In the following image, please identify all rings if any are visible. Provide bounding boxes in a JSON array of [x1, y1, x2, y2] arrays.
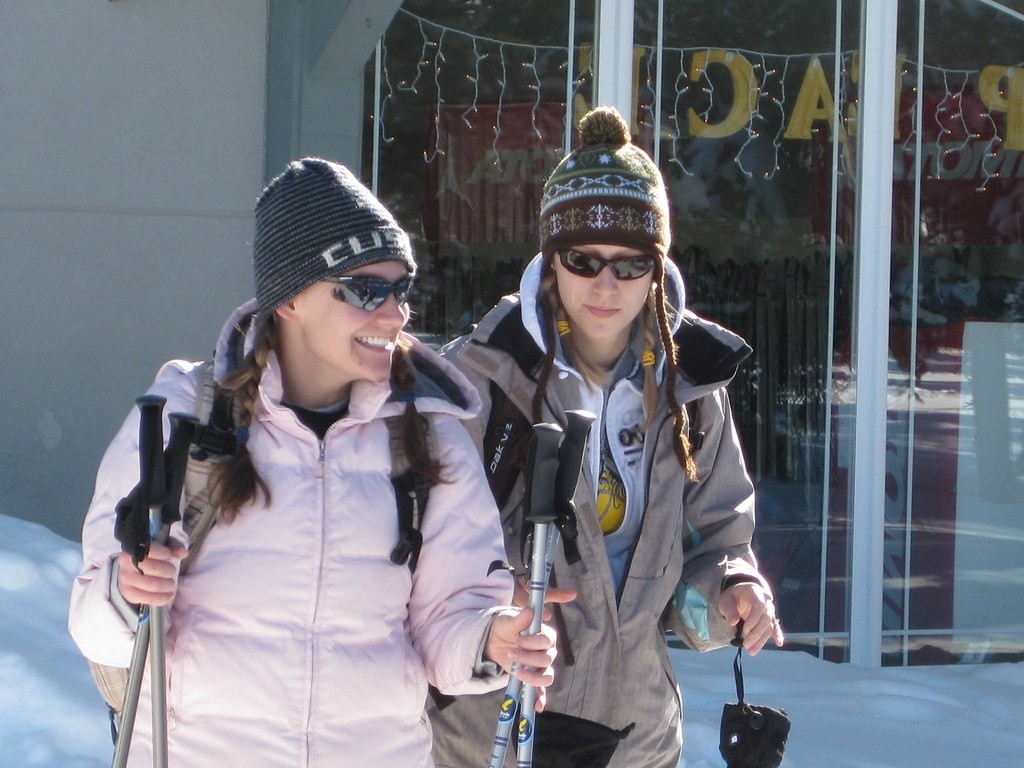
[[771, 616, 780, 630]]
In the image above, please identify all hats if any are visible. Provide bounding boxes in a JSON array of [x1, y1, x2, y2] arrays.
[[505, 106, 698, 482], [253, 156, 417, 317]]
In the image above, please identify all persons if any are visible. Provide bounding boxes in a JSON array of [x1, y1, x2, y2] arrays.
[[69, 157, 558, 768], [428, 106, 785, 768]]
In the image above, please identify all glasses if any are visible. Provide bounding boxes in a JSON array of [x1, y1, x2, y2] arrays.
[[320, 274, 412, 311], [558, 248, 655, 281]]
